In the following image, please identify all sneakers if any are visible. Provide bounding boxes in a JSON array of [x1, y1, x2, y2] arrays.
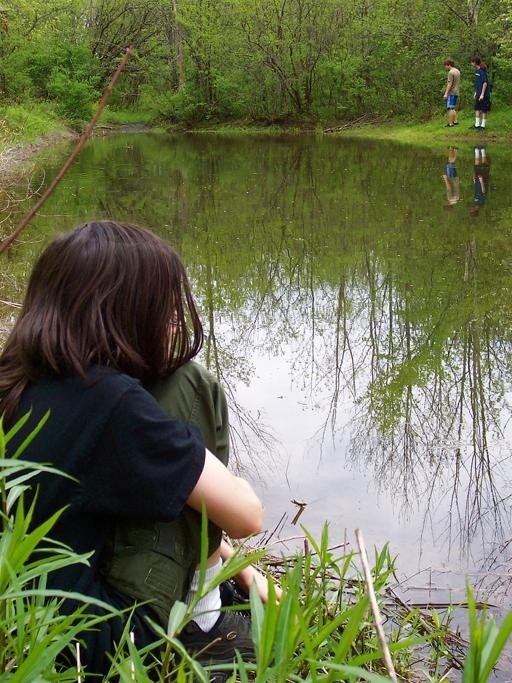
[[468, 125, 486, 131], [444, 121, 459, 128], [179, 579, 300, 677]]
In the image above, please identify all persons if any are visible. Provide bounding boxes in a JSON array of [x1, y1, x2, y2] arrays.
[[470, 145, 491, 217], [469, 55, 490, 130], [444, 60, 461, 128], [442, 144, 461, 211], [1, 219, 289, 683]]
